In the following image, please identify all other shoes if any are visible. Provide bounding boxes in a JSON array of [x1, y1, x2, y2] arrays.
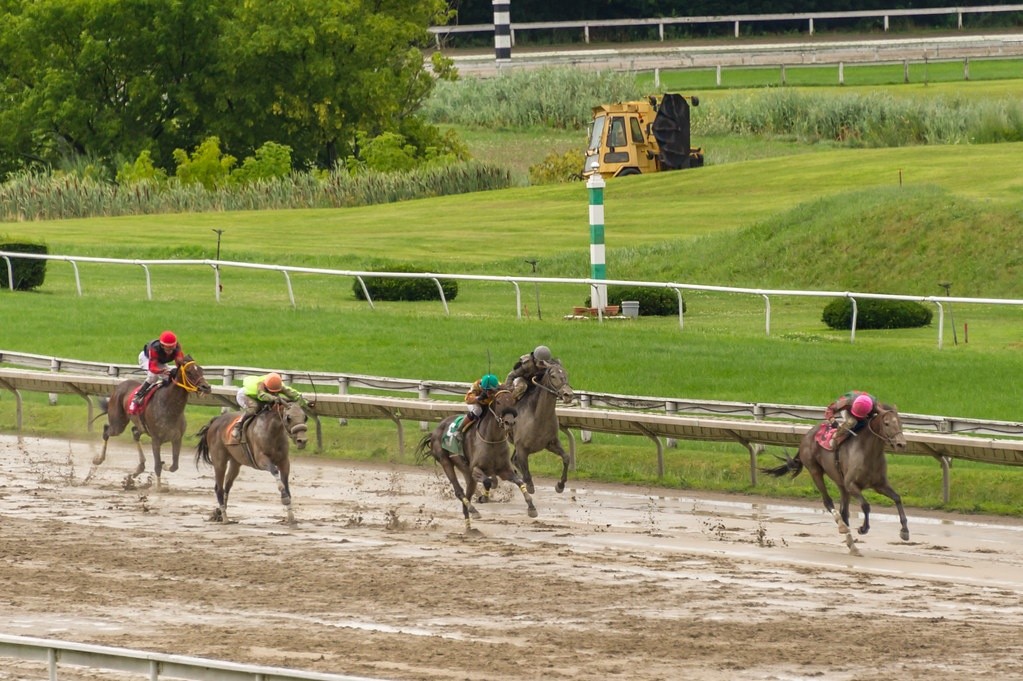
[[453, 431, 464, 442], [829, 438, 837, 451], [134, 394, 140, 404], [232, 428, 241, 440]]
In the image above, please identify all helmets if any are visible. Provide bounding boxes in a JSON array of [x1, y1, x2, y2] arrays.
[[852, 395, 872, 417], [481, 374, 498, 389], [160, 331, 177, 347], [264, 373, 283, 393]]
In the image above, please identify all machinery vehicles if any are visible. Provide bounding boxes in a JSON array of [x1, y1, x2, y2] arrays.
[[576, 93, 705, 182]]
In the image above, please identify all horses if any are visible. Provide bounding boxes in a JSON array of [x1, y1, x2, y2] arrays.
[[90, 354, 211, 492], [414, 384, 539, 520], [192, 396, 309, 524], [759, 402, 910, 540], [479, 357, 574, 504]]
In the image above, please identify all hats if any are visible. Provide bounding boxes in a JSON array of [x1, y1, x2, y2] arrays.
[[533, 346, 552, 363]]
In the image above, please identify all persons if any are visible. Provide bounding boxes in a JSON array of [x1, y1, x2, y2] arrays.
[[506, 345, 551, 402], [452, 374, 510, 440], [233, 372, 311, 439], [131, 330, 185, 405], [824, 389, 879, 450]]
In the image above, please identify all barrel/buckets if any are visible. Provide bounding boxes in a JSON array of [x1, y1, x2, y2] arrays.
[[622, 300, 640, 318]]
